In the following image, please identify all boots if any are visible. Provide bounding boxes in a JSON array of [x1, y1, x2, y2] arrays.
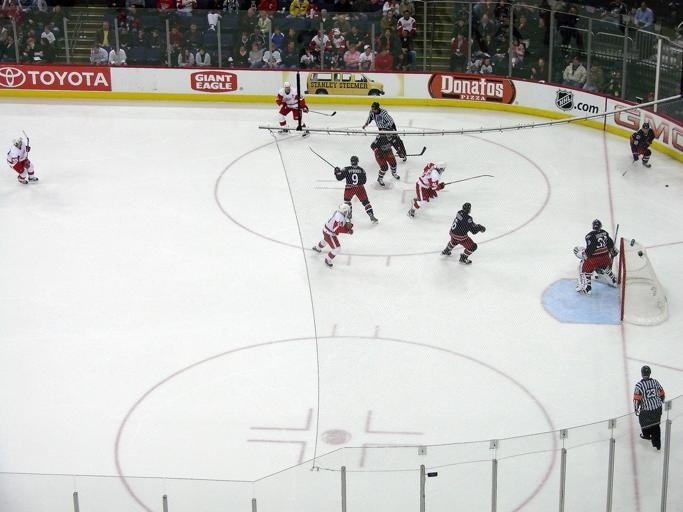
[[576, 283, 591, 294], [441, 247, 451, 255], [407, 208, 414, 217], [458, 253, 472, 263]]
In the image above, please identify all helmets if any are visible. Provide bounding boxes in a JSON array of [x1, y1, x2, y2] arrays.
[[642, 122, 649, 129], [641, 365, 651, 376], [338, 201, 351, 217], [283, 82, 290, 88], [434, 161, 447, 169], [349, 156, 358, 163], [462, 202, 471, 212], [10, 135, 23, 146], [592, 219, 601, 227]]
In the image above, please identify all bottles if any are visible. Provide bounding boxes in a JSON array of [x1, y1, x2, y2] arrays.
[[228, 56, 234, 68]]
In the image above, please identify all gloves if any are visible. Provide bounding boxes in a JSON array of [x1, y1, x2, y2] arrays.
[[633, 154, 638, 161], [302, 107, 309, 113], [573, 247, 588, 261], [279, 102, 286, 109], [25, 146, 30, 152], [438, 182, 444, 189], [334, 167, 341, 174]]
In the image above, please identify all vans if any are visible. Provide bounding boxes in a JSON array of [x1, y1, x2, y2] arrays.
[[305, 72, 385, 97]]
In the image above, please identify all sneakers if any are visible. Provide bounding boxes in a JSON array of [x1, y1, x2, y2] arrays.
[[27, 176, 37, 181], [312, 246, 321, 252], [369, 215, 377, 222], [377, 157, 406, 186], [642, 161, 651, 167], [278, 129, 288, 133], [302, 125, 308, 137], [17, 176, 27, 184], [324, 257, 333, 266], [640, 433, 650, 439]]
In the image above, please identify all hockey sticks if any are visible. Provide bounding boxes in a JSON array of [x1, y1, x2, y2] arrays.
[[591, 224, 619, 280], [22, 131, 29, 158], [444, 175, 493, 184], [622, 160, 636, 175], [394, 147, 426, 156], [308, 110, 336, 116], [306, 144, 335, 168]]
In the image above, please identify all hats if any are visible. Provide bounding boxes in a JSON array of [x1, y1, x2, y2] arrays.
[[371, 102, 379, 108]]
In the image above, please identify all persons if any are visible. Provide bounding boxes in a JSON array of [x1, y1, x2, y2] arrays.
[[405, 160, 445, 221], [574, 220, 617, 294], [628, 122, 655, 170], [632, 364, 665, 453], [5, 136, 38, 185], [440, 202, 486, 265], [87, 1, 418, 69], [368, 127, 400, 188], [275, 82, 310, 136], [0, 1, 72, 60], [332, 156, 378, 223], [361, 102, 407, 163], [447, 1, 681, 99], [311, 204, 350, 268]]
